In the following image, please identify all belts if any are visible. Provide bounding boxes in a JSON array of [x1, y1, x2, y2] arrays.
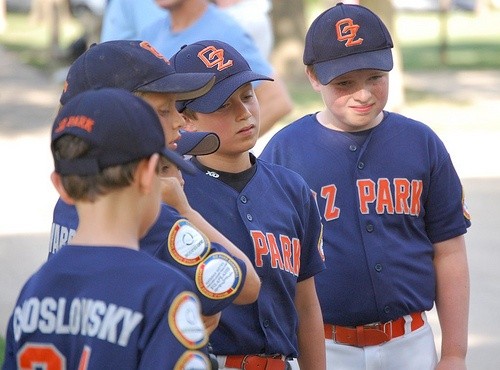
[[225, 354, 286, 370], [324, 310, 424, 346]]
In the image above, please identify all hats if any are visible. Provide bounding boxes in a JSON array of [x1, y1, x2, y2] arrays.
[[51, 85, 198, 177], [169, 39, 274, 113], [60, 40, 216, 105], [303, 2, 394, 86], [174, 127, 220, 156]]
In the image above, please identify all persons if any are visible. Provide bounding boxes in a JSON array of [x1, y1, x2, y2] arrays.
[[167, 38, 327, 370], [135, 0, 293, 139], [98, 0, 275, 61], [46, 39, 261, 317], [1, 87, 211, 370], [257, 2, 472, 370], [160, 152, 222, 336]]
